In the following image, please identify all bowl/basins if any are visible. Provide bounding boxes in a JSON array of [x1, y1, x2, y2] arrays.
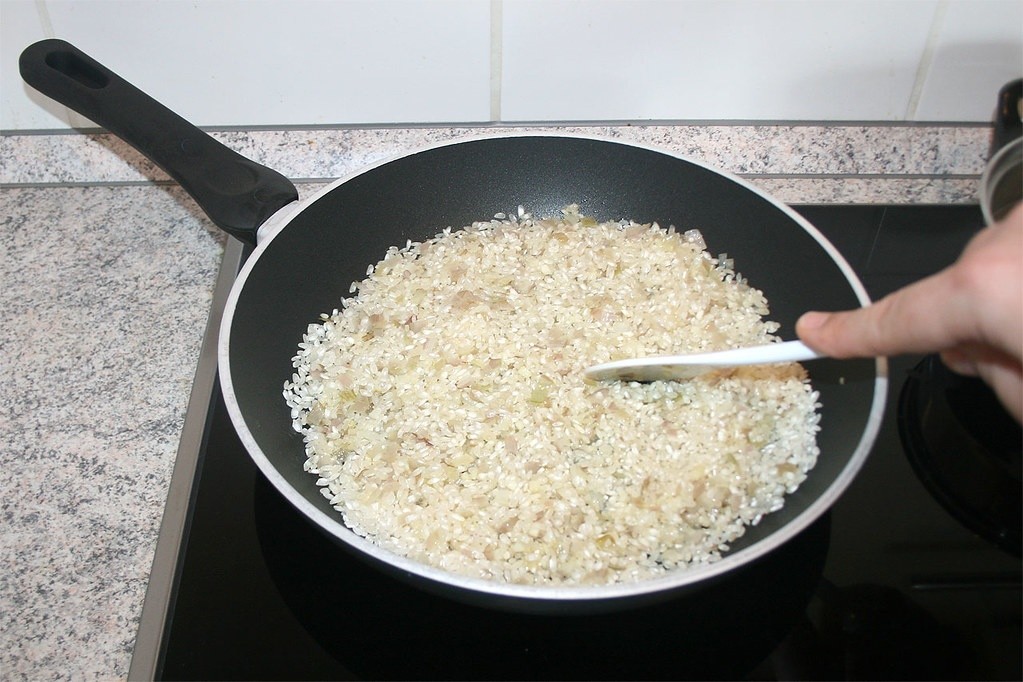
[[981, 136, 1023, 228]]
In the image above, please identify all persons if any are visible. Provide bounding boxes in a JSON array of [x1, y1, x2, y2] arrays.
[[795, 199, 1023, 424]]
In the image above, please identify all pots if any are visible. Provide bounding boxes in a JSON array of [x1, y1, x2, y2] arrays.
[[17, 37, 889, 619]]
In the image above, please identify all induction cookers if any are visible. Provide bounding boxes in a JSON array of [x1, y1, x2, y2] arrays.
[[126, 202, 1022, 682]]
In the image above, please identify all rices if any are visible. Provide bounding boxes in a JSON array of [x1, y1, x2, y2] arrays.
[[280, 197, 828, 586]]
